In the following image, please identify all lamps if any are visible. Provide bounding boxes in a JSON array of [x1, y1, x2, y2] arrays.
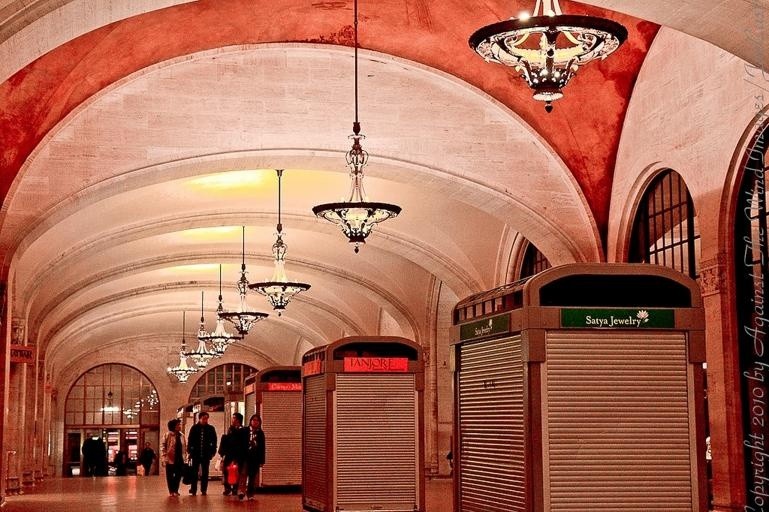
[[166, 166, 311, 383], [466, 1, 627, 115], [312, 2, 404, 254]]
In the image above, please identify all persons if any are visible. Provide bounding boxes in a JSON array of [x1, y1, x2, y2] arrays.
[[218, 411, 245, 496], [159, 419, 190, 497], [114, 445, 129, 476], [186, 411, 218, 496], [139, 442, 158, 476], [231, 414, 266, 501]]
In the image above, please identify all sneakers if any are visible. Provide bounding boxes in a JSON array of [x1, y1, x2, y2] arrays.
[[170, 489, 206, 496], [223, 490, 255, 500]]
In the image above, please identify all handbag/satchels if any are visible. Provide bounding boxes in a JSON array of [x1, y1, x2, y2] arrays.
[[227, 461, 239, 486], [183, 466, 191, 486], [180, 464, 188, 475], [218, 435, 225, 456]]
